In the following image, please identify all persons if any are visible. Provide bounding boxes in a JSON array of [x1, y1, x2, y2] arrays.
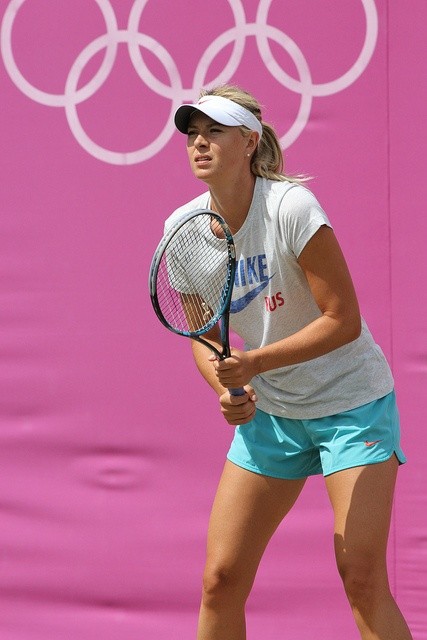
[[161, 80, 417, 639]]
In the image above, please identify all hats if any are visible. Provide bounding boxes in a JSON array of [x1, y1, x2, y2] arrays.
[[175, 95, 263, 143]]
